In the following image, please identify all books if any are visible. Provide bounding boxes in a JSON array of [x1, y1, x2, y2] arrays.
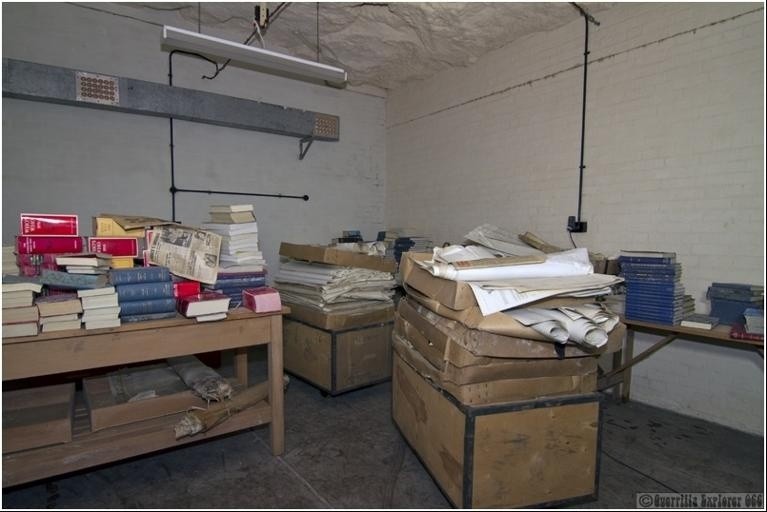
[[378, 230, 434, 272], [679, 313, 720, 331], [327, 230, 364, 247], [707, 281, 764, 336], [592, 248, 696, 326], [2, 203, 282, 338]]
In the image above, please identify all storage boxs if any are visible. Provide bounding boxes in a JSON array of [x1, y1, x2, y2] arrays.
[[283, 316, 394, 397], [389, 350, 604, 509]]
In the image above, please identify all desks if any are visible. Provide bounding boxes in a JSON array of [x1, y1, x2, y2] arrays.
[[598, 314, 764, 404], [2, 306, 292, 490]]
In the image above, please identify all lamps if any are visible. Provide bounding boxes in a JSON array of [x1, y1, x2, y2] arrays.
[[160, 24, 348, 85]]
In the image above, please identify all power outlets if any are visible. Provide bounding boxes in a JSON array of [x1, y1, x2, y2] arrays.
[[567, 222, 587, 232]]
